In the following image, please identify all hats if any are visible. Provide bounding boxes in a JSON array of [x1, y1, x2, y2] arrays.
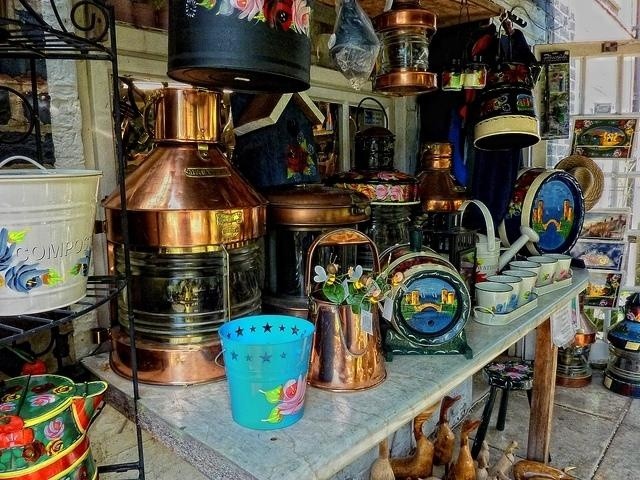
[[554, 155, 605, 213]]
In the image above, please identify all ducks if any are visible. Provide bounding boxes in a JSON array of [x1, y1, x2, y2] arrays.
[[370, 397, 483, 480]]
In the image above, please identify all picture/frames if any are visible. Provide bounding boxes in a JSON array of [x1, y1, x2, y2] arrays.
[[583, 273, 623, 310], [578, 206, 631, 241], [571, 238, 628, 273], [568, 115, 639, 157]]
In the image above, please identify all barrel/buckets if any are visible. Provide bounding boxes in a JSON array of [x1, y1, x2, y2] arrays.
[[0, 155, 103, 317], [373, 245, 473, 347], [215, 315, 316, 430], [102, 145, 269, 386]]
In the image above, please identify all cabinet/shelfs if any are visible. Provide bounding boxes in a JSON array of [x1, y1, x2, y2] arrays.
[[570, 130, 640, 342], [0, 0, 145, 480]]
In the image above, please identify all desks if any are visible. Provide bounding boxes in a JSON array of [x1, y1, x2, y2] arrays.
[[78, 268, 590, 480]]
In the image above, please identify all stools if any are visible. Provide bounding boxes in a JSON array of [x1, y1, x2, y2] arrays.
[[471, 359, 552, 463]]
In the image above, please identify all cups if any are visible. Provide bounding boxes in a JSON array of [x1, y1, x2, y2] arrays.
[[474, 253, 573, 314]]
